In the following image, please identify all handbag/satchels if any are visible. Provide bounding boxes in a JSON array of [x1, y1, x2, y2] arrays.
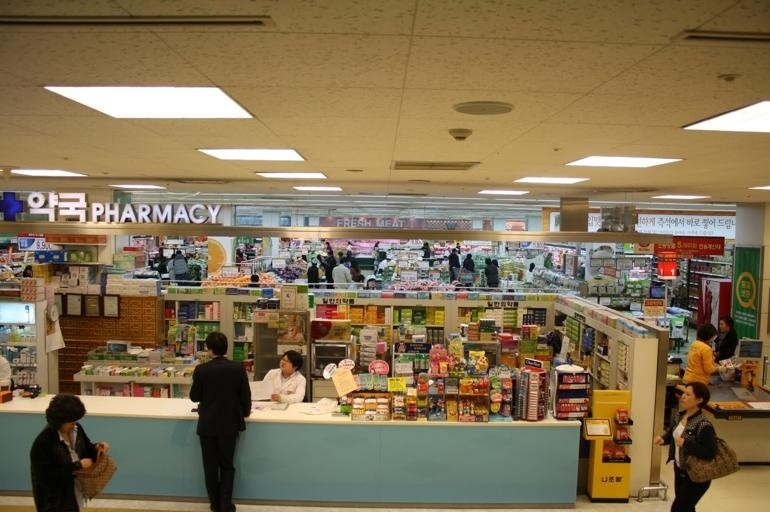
[[73, 448, 117, 500], [686, 419, 740, 483]]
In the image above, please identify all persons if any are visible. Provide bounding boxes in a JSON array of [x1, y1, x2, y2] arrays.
[[0, 357, 12, 393], [525, 263, 544, 285], [301, 240, 500, 291], [714, 316, 738, 365], [248, 350, 308, 404], [237, 242, 260, 287], [544, 252, 554, 271], [29, 393, 110, 512], [654, 381, 717, 512], [149, 242, 199, 286], [190, 331, 252, 512], [680, 322, 729, 389]]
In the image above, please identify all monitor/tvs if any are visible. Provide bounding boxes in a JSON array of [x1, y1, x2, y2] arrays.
[[735, 337, 763, 362], [650, 286, 665, 299]]
